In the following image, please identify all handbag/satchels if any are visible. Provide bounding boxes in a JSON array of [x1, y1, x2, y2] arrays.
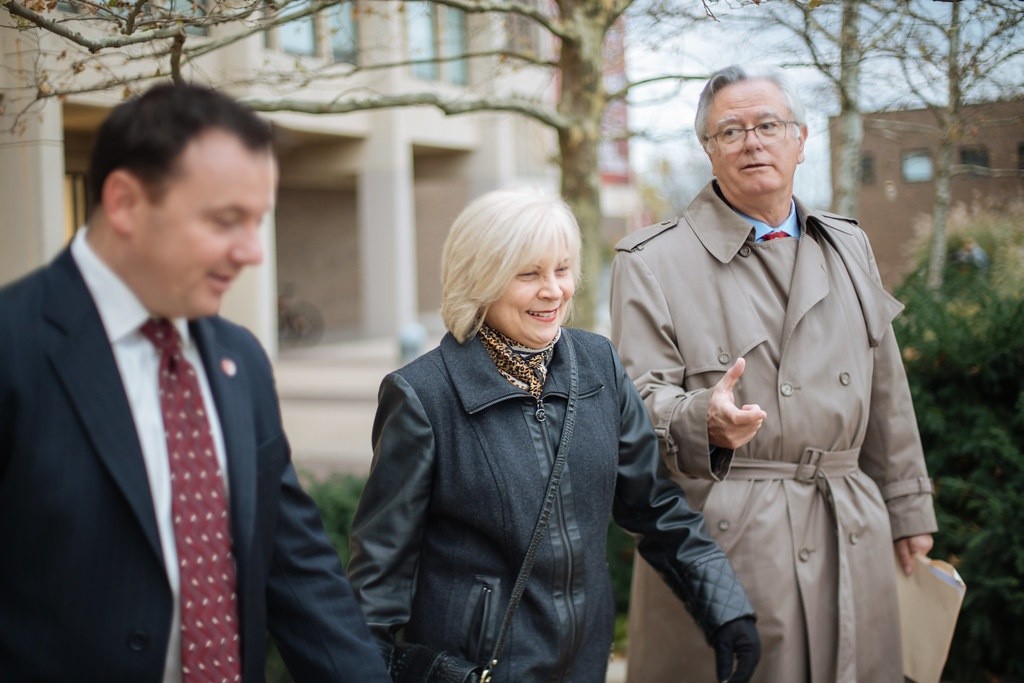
[[386, 641, 490, 683]]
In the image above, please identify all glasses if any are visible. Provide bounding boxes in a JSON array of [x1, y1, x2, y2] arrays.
[[707, 119, 800, 154]]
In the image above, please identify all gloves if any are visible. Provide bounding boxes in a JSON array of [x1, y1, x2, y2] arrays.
[[713, 612, 762, 682]]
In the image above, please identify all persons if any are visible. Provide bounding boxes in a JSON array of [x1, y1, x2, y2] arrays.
[[347, 184, 763, 682], [612, 67, 937, 683], [2, 80, 392, 683]]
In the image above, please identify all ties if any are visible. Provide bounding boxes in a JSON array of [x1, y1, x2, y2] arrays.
[[142, 318, 243, 682]]
